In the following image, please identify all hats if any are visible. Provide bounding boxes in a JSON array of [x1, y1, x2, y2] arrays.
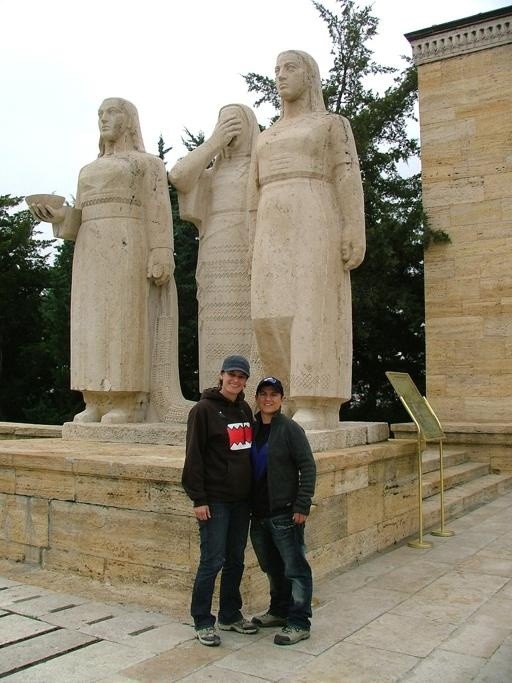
[[256, 377, 283, 395], [221, 355, 250, 377]]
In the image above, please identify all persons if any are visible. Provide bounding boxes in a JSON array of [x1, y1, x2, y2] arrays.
[[180, 354, 259, 646], [250, 375, 317, 645], [168, 103, 264, 393], [23, 96, 178, 424], [246, 49, 367, 428]]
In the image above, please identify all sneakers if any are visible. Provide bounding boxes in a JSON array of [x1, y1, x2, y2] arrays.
[[274, 624, 310, 645], [195, 626, 220, 646], [217, 618, 259, 634], [251, 613, 289, 627]]
